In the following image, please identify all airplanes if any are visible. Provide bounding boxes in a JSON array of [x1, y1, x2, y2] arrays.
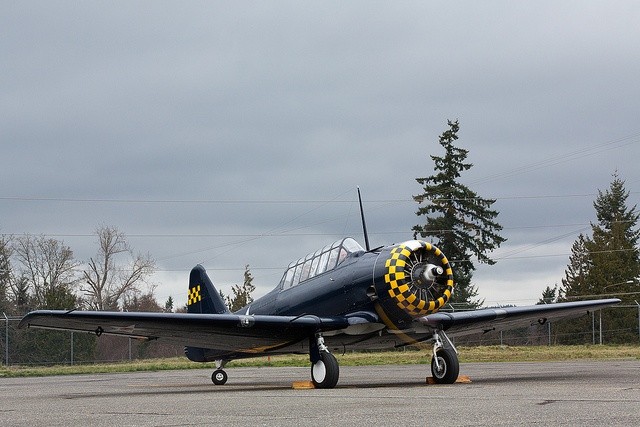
[[19, 188, 621, 388]]
